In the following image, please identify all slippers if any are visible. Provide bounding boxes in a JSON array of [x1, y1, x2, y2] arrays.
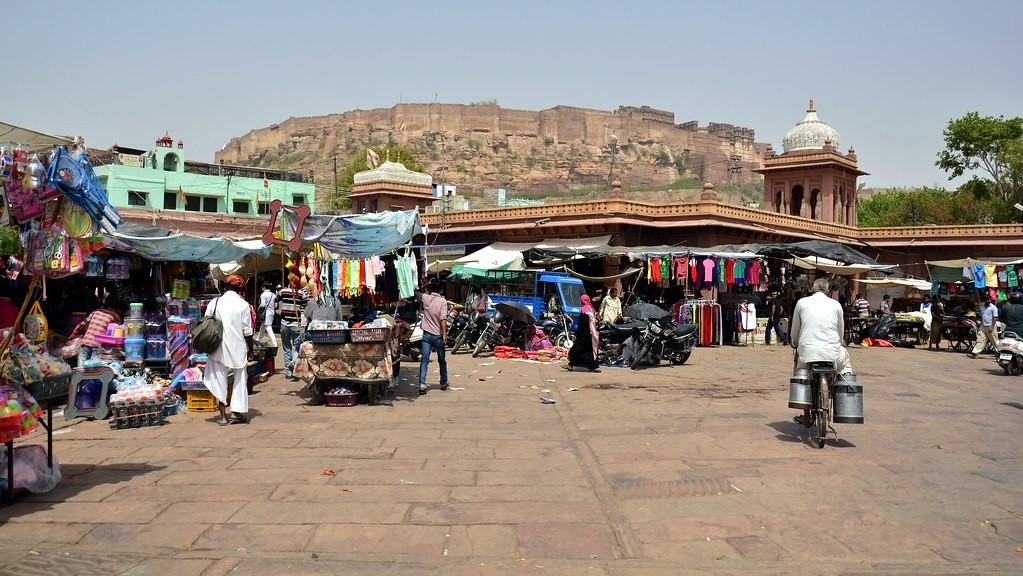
[[228, 415, 247, 421], [216, 418, 228, 426], [794, 415, 811, 429]]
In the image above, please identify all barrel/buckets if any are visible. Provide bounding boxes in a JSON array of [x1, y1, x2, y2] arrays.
[[130, 303, 143, 319], [788, 367, 813, 410], [832, 372, 864, 424]]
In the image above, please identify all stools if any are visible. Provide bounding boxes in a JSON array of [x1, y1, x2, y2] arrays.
[[108, 399, 163, 429]]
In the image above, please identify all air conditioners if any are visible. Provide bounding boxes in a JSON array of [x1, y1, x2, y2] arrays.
[[426, 204, 439, 213]]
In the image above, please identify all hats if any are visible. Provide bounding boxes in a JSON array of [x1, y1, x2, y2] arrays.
[[224, 274, 245, 290]]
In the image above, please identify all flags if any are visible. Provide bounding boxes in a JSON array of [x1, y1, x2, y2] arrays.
[[366, 148, 380, 170], [396, 117, 406, 136]]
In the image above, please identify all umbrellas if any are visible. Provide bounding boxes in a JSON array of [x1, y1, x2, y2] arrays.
[[495, 300, 537, 336], [623, 303, 671, 320]]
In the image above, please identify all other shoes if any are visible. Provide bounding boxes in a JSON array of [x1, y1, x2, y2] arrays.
[[561, 365, 573, 371], [966, 353, 977, 359], [783, 338, 789, 346], [288, 362, 300, 381], [286, 375, 293, 379], [589, 369, 602, 373], [441, 380, 450, 390], [419, 386, 431, 395], [760, 342, 772, 346]]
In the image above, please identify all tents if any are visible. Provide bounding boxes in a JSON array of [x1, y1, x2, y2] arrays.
[[429, 234, 1023, 315]]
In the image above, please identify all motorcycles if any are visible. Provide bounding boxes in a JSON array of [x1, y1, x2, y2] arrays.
[[631, 315, 699, 370], [402, 302, 576, 357]]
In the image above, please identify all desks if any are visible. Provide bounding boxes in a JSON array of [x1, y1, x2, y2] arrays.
[[5, 391, 68, 505], [121, 359, 172, 379]]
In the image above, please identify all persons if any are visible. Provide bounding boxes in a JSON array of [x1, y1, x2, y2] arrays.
[[201, 274, 253, 426], [348, 286, 377, 328], [66, 280, 128, 368], [940, 296, 971, 318], [275, 284, 281, 295], [852, 292, 871, 329], [763, 292, 790, 346], [0, 284, 20, 335], [560, 294, 601, 373], [620, 327, 652, 368], [919, 294, 947, 351], [468, 286, 480, 309], [880, 295, 891, 318], [966, 294, 1023, 359], [256, 282, 278, 346], [548, 296, 560, 315], [598, 288, 623, 325], [413, 281, 450, 395], [790, 277, 852, 428], [527, 323, 553, 351], [275, 282, 343, 380]]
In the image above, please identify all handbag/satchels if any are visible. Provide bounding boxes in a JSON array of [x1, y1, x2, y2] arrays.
[[24, 301, 48, 342], [191, 315, 223, 355], [256, 306, 266, 322], [26, 219, 84, 279]]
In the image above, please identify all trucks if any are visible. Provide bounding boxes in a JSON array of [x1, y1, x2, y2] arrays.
[[484, 268, 586, 333]]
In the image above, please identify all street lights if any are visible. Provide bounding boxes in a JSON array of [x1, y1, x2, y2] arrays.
[[855, 181, 867, 228], [609, 135, 618, 191]]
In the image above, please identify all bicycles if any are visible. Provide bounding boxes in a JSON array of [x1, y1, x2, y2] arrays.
[[791, 343, 848, 448]]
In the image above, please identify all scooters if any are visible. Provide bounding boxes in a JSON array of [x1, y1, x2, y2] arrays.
[[994, 330, 1023, 376]]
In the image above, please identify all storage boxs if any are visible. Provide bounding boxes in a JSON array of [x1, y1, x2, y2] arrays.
[[324, 385, 367, 406], [94, 334, 125, 345], [347, 327, 390, 342], [164, 345, 278, 414], [307, 328, 349, 344], [20, 372, 76, 401]]
